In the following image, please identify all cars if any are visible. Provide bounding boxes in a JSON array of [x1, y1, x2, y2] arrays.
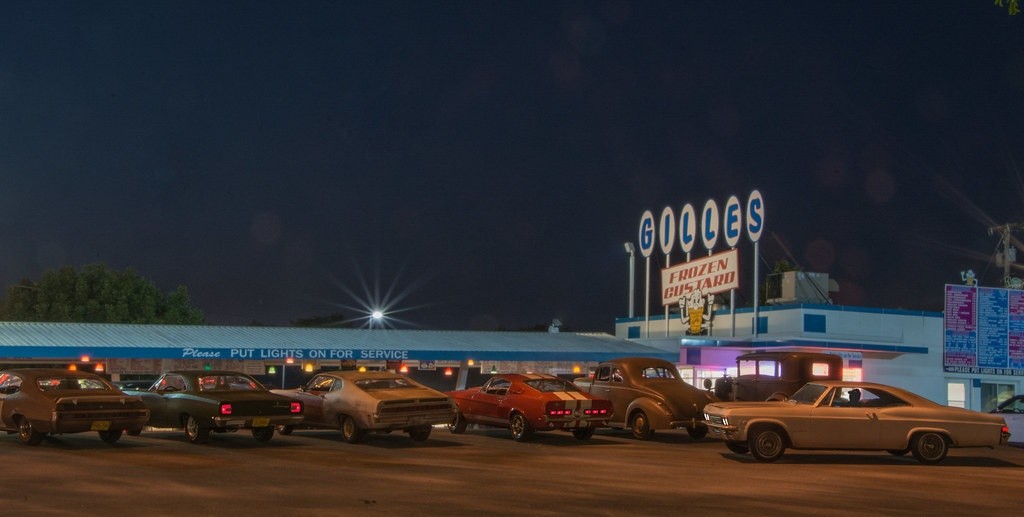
[[703, 380, 1013, 465], [0, 366, 151, 445], [443, 373, 615, 443], [271, 371, 460, 443], [120, 370, 305, 444], [703, 351, 844, 401], [985, 396, 1024, 448], [573, 357, 720, 441]]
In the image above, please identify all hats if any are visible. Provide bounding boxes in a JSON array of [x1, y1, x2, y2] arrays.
[[848, 389, 861, 397]]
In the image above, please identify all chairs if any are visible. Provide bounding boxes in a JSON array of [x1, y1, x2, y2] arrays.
[[216, 383, 231, 391], [357, 381, 390, 389], [58, 379, 80, 389]]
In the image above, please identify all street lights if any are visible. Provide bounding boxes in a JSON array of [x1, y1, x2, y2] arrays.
[[369, 310, 383, 330]]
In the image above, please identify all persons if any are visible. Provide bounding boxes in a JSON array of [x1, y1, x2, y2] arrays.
[[842, 389, 861, 406]]
